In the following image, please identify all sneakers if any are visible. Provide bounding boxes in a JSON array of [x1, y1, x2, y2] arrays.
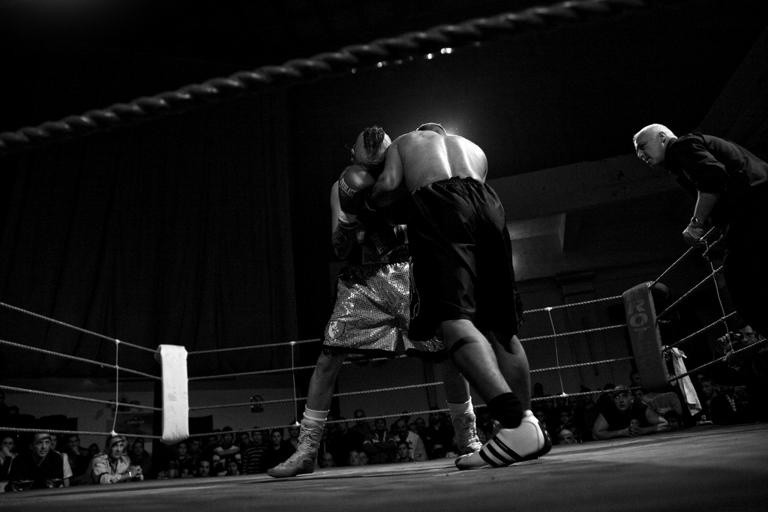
[[453, 408, 553, 470]]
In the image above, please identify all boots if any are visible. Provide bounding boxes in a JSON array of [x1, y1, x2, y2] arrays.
[[450, 409, 484, 456], [266, 420, 325, 478]]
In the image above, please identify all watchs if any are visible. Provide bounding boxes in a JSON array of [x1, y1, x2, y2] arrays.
[[690, 217, 707, 230]]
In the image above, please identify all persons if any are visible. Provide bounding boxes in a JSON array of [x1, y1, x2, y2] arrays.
[[0, 365, 767, 490], [267, 130, 483, 476], [633, 125, 768, 340], [374, 122, 553, 466]]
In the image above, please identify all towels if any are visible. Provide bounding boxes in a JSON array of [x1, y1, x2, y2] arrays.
[[670, 346, 703, 416]]
